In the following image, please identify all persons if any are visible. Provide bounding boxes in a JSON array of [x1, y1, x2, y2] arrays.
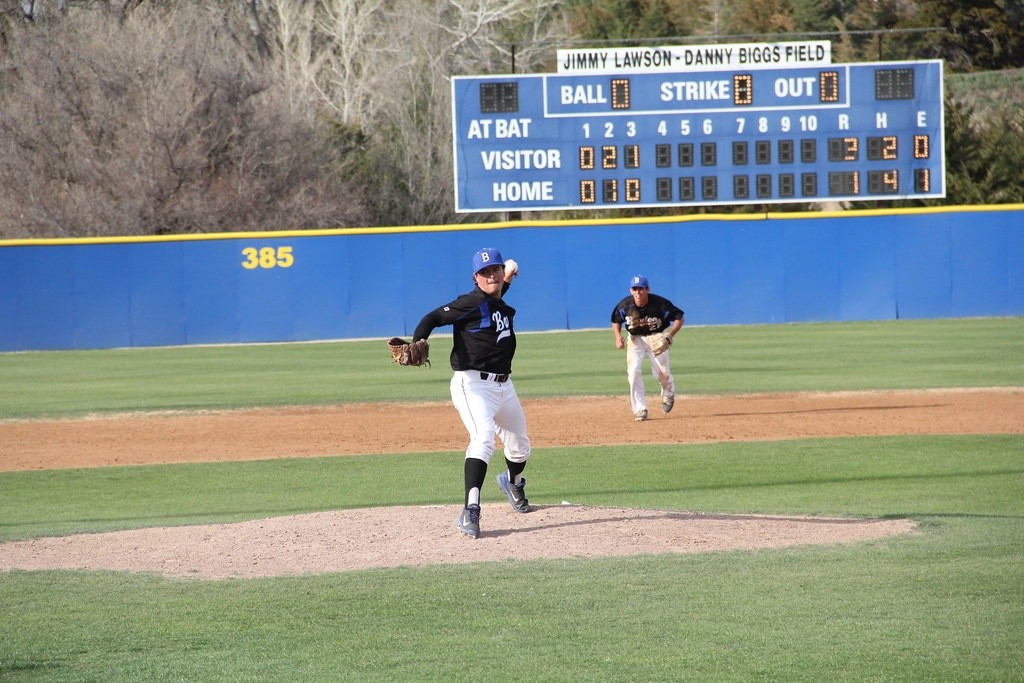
[[611, 273, 684, 422], [387, 248, 531, 539]]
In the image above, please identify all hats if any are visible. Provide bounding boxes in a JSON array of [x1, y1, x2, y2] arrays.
[[630, 274, 650, 288], [472, 247, 506, 275]]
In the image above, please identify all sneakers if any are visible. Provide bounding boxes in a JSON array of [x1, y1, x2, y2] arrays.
[[634, 409, 648, 421], [662, 395, 674, 412], [497, 469, 533, 514], [457, 502, 482, 539]]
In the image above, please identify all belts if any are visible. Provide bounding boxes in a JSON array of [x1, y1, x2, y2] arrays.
[[480, 372, 509, 382]]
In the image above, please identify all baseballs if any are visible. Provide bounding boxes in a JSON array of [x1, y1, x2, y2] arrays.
[[504, 260, 518, 274]]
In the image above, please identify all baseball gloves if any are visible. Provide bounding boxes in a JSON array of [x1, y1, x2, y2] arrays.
[[386, 337, 432, 371], [647, 332, 673, 357]]
